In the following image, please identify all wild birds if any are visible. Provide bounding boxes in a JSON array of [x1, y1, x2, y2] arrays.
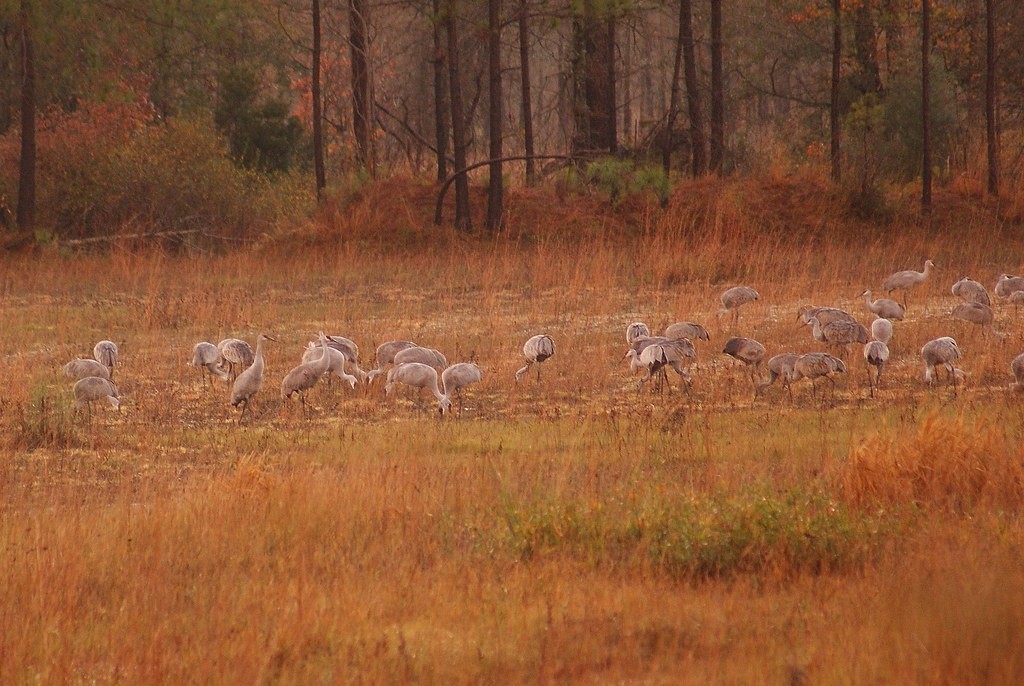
[[58, 340, 123, 428], [617, 321, 710, 405], [280, 329, 483, 423], [185, 333, 280, 425], [514, 333, 558, 386], [719, 259, 1024, 405]]
